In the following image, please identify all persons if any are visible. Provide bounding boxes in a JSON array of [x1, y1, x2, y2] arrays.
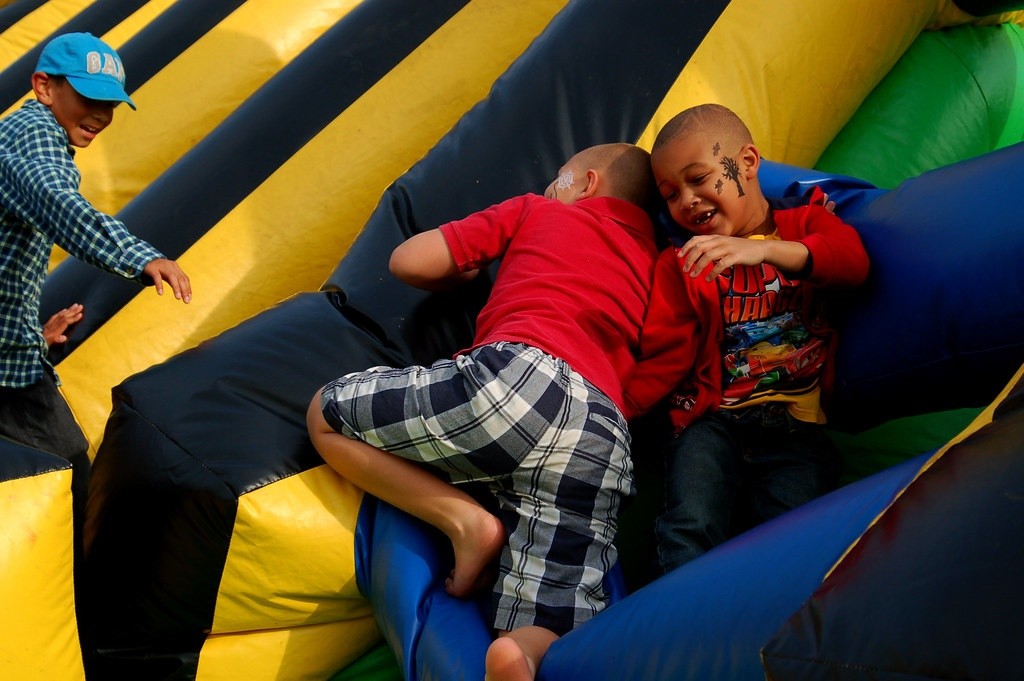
[[0, 32, 191, 550], [627, 103, 870, 569], [307, 143, 834, 681]]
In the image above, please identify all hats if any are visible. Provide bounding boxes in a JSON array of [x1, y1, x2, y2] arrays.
[[36, 32, 137, 111]]
